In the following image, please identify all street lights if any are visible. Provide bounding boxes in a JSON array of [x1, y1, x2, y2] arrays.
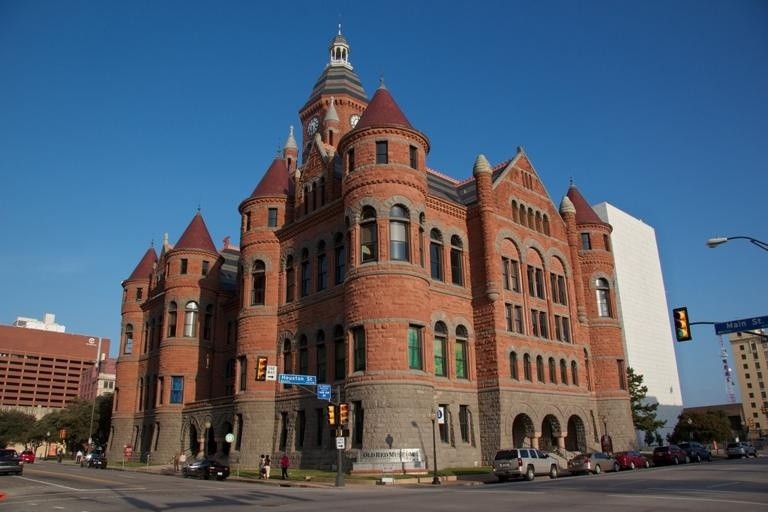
[[430, 409, 441, 485], [601, 415, 608, 456], [707, 236, 768, 251], [688, 418, 693, 439]]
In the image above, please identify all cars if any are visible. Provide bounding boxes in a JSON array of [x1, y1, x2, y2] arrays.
[[618, 449, 653, 469], [184, 461, 229, 480], [20, 451, 35, 464], [0, 449, 23, 476], [80, 454, 106, 470], [567, 452, 620, 474]]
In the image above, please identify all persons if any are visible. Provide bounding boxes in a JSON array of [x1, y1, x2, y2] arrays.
[[175, 451, 180, 471], [280, 453, 289, 479], [180, 452, 186, 468], [259, 454, 272, 480], [199, 455, 208, 479], [56, 445, 82, 463]]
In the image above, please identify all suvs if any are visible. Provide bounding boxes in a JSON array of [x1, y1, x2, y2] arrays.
[[492, 448, 559, 481]]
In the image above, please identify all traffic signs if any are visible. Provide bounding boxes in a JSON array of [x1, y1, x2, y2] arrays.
[[278, 374, 317, 386], [715, 316, 768, 335]]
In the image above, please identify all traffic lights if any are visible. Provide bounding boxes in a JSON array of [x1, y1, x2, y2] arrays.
[[256, 358, 267, 380], [670, 308, 690, 342], [341, 403, 349, 425], [326, 406, 335, 425]]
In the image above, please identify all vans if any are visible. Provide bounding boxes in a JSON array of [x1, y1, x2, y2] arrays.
[[680, 441, 714, 462], [654, 445, 690, 465], [726, 441, 758, 459]]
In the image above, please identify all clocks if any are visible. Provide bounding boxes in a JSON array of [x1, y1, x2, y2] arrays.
[[348, 113, 361, 129], [306, 115, 320, 137]]
